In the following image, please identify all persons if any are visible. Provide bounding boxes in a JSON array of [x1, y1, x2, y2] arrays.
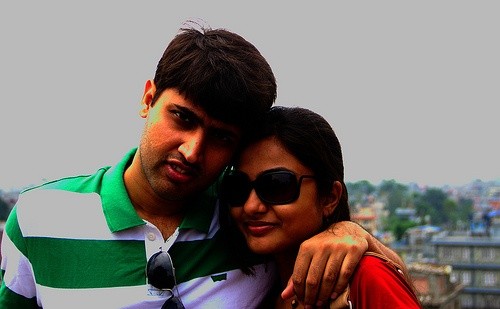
[[0, 16, 410, 309], [216, 106, 421, 309]]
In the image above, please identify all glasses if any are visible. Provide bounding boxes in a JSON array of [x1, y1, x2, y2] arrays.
[[147, 247, 185, 309], [220, 170, 317, 207]]
